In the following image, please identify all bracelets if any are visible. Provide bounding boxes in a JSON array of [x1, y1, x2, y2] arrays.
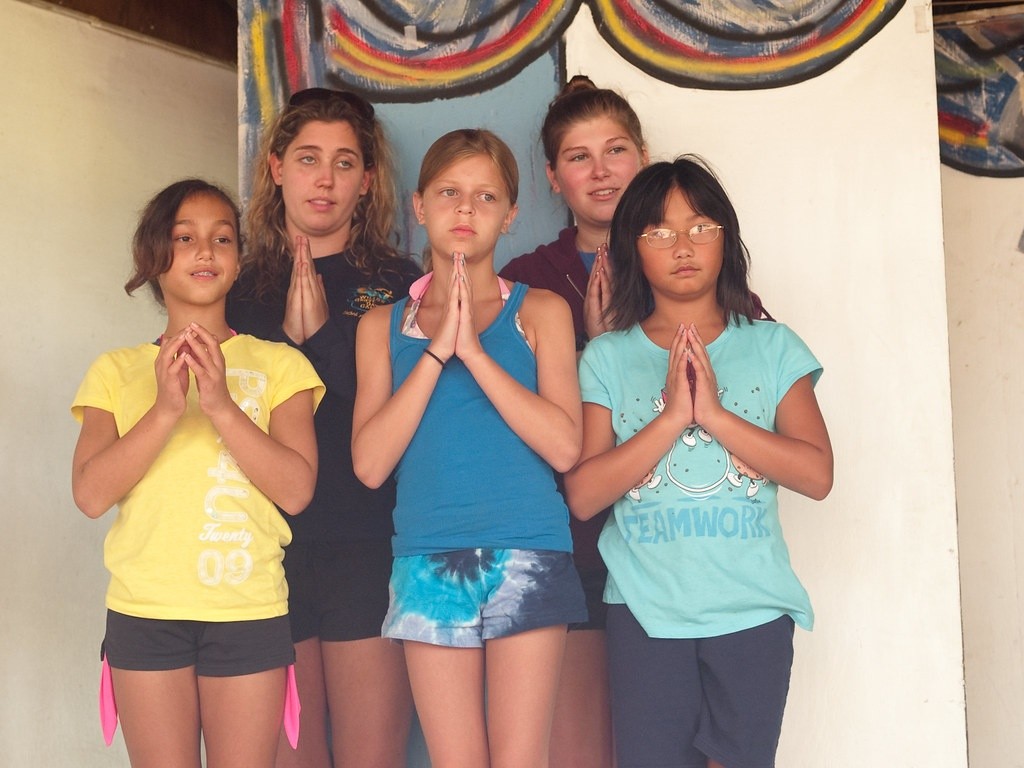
[[423, 349, 445, 369]]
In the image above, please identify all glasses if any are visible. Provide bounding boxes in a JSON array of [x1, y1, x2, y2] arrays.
[[636, 223, 724, 249]]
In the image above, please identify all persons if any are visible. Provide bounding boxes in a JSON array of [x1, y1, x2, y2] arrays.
[[351, 129, 589, 768], [495, 75, 762, 768], [69, 179, 327, 768], [562, 159, 834, 768], [225, 86, 425, 768]]
